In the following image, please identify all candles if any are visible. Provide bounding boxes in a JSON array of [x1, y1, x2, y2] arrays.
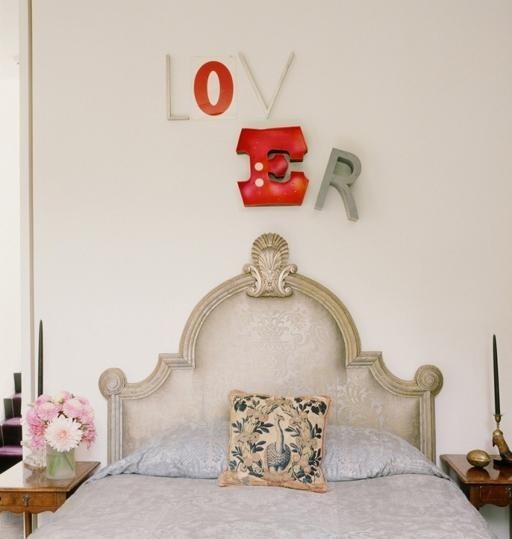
[[493, 334, 501, 416]]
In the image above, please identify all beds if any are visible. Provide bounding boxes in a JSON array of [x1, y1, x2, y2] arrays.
[[20, 232, 499, 539]]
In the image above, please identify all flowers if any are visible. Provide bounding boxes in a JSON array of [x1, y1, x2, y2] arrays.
[[26, 392, 97, 471]]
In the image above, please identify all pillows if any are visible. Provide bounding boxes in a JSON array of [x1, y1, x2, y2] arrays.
[[219, 388, 332, 495], [85, 420, 230, 481], [324, 424, 448, 480]]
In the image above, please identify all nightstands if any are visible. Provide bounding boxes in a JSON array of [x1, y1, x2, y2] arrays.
[[437, 454, 511, 539], [0, 456, 99, 539]]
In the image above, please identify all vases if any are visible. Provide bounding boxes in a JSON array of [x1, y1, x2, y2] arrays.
[[46, 445, 75, 480]]
